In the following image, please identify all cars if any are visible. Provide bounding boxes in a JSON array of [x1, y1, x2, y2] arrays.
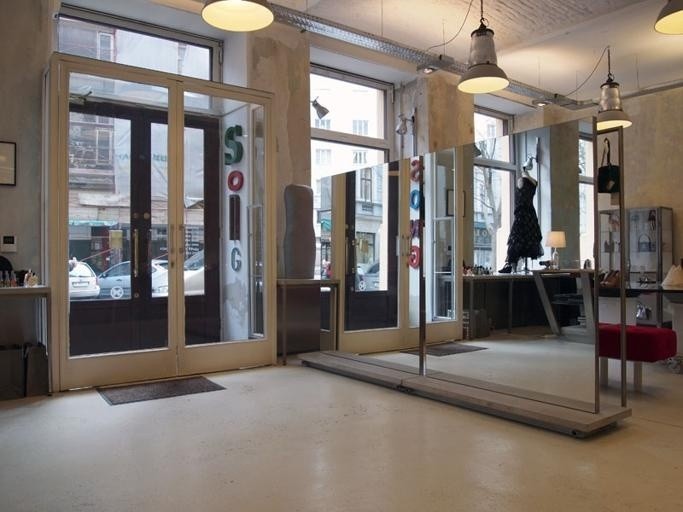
[[357, 261, 380, 291], [69, 249, 204, 297]]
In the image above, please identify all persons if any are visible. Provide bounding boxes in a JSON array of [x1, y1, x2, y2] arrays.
[[505, 169, 545, 264]]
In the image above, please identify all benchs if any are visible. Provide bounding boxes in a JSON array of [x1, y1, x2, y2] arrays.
[[599, 322, 677, 392]]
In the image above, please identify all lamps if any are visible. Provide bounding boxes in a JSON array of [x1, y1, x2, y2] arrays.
[[654, 0, 683, 36], [200, 0, 274, 33], [457, 0, 510, 95], [395, 113, 416, 136], [310, 99, 329, 119], [595, 45, 632, 132], [545, 231, 567, 270]]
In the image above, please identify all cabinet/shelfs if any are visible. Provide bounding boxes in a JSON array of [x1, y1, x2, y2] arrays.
[[599, 206, 675, 328]]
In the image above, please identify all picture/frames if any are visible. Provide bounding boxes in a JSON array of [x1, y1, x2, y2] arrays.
[[0, 140, 17, 186], [446, 190, 465, 218]]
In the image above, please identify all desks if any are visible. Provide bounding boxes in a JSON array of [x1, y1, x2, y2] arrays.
[[438, 272, 570, 341], [0, 283, 53, 397]]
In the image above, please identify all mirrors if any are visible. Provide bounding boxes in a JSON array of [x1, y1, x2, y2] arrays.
[[320, 116, 600, 413]]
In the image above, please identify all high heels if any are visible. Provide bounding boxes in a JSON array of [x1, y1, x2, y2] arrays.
[[599, 269, 620, 289]]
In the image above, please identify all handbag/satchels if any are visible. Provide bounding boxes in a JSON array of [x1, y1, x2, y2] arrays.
[[635, 301, 653, 321], [596, 160, 623, 194], [636, 233, 655, 252]]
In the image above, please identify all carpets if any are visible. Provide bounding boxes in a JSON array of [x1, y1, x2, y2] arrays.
[[400, 342, 488, 357], [95, 375, 227, 406]]
